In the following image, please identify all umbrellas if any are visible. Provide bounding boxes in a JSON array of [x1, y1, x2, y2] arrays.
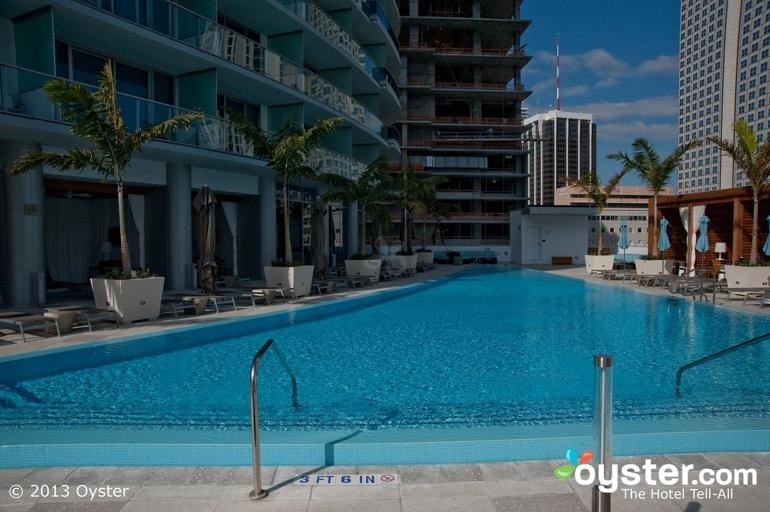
[[656, 216, 672, 274], [192, 184, 218, 297], [615, 222, 631, 273], [696, 215, 710, 267], [762, 212, 770, 256]]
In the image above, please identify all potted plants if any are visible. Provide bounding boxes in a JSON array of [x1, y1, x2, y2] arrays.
[[8, 58, 204, 326], [223, 102, 344, 300], [557, 116, 769, 296], [319, 146, 448, 285]]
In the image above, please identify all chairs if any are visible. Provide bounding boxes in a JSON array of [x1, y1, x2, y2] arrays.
[[722, 288, 770, 307], [312, 278, 348, 296], [1, 278, 298, 341]]
